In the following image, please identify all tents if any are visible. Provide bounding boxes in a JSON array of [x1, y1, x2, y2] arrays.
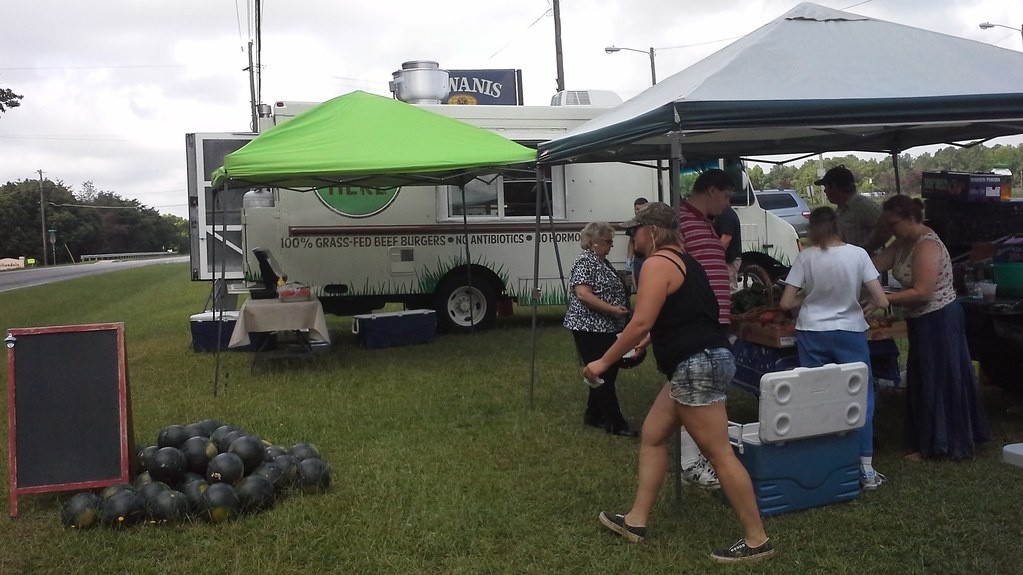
[[210, 90, 583, 399], [536, 1, 1022, 501]]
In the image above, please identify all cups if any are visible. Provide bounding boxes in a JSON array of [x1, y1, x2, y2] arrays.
[[982, 284, 997, 303]]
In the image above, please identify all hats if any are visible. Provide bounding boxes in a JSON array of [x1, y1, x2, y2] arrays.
[[814, 164, 854, 188], [619, 202, 677, 229]]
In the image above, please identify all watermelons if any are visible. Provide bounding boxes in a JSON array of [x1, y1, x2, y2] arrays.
[[60, 419, 328, 530]]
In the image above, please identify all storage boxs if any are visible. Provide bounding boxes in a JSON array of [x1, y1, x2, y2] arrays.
[[921, 168, 1023, 242], [352, 309, 437, 348], [716, 315, 909, 516], [191, 311, 265, 353]]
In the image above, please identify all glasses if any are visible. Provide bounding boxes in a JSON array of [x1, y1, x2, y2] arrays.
[[599, 239, 613, 245], [888, 217, 905, 228]]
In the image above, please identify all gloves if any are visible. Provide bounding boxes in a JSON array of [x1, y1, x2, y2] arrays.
[[625, 259, 634, 270]]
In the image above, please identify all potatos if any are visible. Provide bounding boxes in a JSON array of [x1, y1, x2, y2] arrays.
[[749, 310, 795, 341]]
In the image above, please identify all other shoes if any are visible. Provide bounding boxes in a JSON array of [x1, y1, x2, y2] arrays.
[[606, 428, 638, 438], [584, 418, 603, 429]]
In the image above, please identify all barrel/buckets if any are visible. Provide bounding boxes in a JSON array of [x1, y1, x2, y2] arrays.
[[989, 263, 1023, 297]]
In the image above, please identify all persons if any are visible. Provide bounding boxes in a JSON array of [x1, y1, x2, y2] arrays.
[[625, 197, 648, 292], [707, 205, 742, 293], [681, 170, 737, 489], [871, 195, 991, 463], [780, 206, 889, 490], [563, 222, 639, 437], [815, 164, 893, 285], [583, 202, 775, 564]]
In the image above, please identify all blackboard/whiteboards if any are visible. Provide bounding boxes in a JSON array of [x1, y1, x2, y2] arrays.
[[7, 321, 128, 490]]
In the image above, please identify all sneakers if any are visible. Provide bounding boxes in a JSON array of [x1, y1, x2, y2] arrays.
[[711, 537, 775, 562], [861, 470, 882, 490], [600, 511, 647, 542]]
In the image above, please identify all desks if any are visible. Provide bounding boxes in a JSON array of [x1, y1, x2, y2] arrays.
[[228, 294, 332, 375], [724, 338, 900, 450]]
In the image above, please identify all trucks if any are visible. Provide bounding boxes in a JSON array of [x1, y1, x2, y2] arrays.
[[185, 90, 805, 333]]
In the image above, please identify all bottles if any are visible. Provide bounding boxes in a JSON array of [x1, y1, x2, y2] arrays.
[[964, 269, 974, 296]]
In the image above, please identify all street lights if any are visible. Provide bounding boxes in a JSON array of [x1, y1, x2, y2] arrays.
[[605, 45, 656, 87], [980, 22, 1023, 44]]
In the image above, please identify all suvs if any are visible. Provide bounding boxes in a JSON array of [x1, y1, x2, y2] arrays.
[[755, 188, 811, 238]]
